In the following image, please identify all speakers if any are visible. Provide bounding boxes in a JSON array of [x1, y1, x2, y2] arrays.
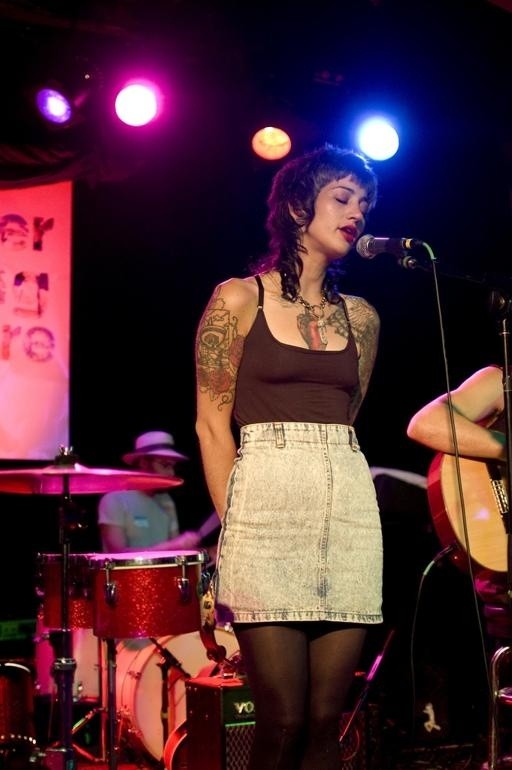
[[184, 675, 369, 770]]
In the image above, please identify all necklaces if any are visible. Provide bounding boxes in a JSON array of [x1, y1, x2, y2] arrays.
[[290, 289, 329, 348]]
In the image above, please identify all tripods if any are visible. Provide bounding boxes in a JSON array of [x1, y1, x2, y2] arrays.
[[28, 633, 162, 770]]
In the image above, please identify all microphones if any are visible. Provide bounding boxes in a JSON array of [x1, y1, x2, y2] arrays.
[[357, 234, 423, 260]]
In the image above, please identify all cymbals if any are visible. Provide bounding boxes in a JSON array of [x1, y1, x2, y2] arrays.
[[0, 460, 181, 496]]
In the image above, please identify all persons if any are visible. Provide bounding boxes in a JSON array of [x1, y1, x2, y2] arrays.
[[195, 147, 384, 770], [98, 431, 204, 554], [405, 357, 511, 465]]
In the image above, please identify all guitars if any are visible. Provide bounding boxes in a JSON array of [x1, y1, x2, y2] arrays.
[[426, 428, 512, 581]]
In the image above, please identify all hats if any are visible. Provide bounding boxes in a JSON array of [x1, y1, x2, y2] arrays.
[[120, 431, 190, 468]]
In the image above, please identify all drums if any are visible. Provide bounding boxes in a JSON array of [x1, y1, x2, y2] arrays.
[[90, 547, 203, 643], [35, 610, 102, 704], [36, 548, 97, 636], [114, 621, 239, 765]]
